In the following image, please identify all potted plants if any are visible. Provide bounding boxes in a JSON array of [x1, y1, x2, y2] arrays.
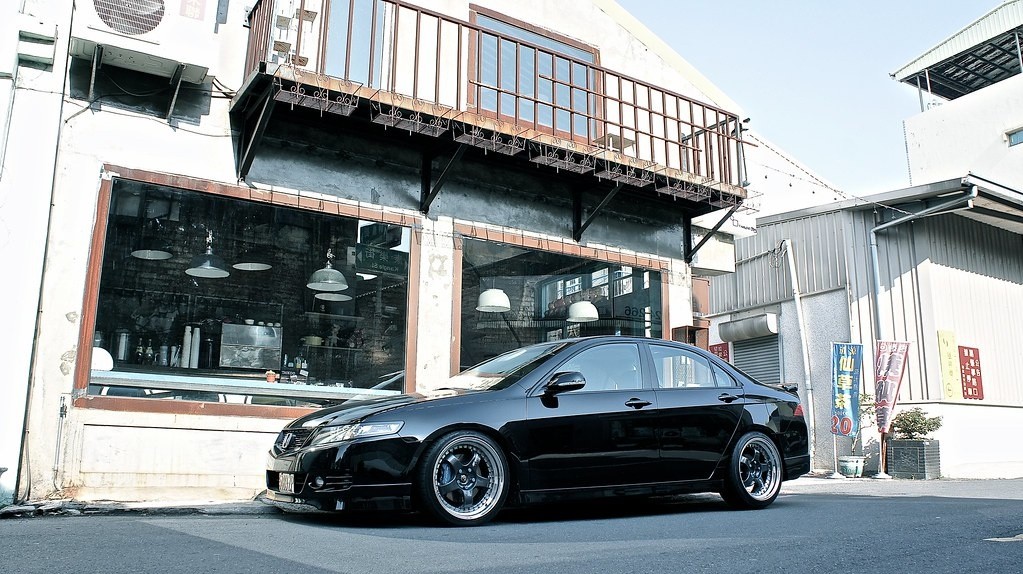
[[885, 407, 944, 480], [837, 393, 878, 478]]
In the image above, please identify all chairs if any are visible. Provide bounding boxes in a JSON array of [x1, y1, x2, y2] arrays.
[[582, 367, 639, 391]]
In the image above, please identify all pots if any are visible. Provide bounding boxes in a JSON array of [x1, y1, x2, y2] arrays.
[[300, 333, 325, 346]]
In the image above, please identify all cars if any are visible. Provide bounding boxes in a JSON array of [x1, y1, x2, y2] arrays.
[[267, 336, 810, 524]]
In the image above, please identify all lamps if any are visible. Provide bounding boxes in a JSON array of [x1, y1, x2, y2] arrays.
[[566, 285, 600, 323], [476, 273, 511, 313], [131, 210, 175, 261], [314, 292, 353, 302], [185, 224, 230, 278], [306, 242, 350, 292], [232, 228, 273, 271]]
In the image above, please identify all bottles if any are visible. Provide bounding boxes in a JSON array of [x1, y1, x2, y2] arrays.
[[134, 337, 154, 366]]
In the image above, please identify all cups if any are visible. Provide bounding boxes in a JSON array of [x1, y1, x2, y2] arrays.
[[180, 324, 201, 369]]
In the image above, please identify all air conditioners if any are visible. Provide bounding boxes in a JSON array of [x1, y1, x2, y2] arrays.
[[69, 0, 221, 85]]
